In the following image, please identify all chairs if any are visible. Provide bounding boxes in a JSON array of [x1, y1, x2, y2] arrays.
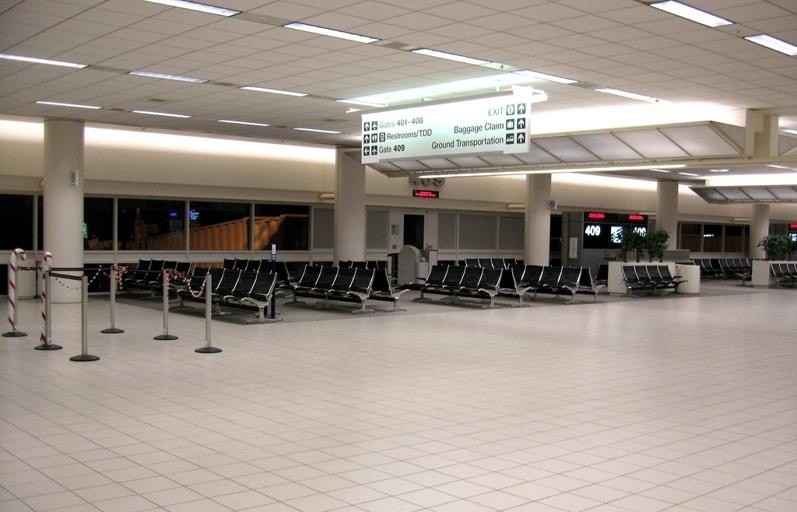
[[621, 264, 688, 300], [395, 257, 608, 310], [98, 256, 412, 323], [694, 258, 796, 289]]
